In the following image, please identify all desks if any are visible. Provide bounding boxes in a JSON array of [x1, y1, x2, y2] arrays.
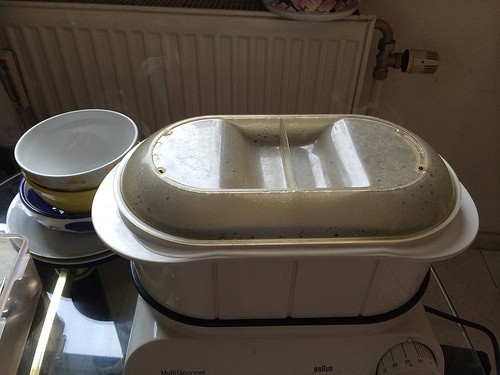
[[1, 191, 499, 375]]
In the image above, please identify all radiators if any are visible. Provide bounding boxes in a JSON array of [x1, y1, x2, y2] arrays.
[[1, 1, 438, 137]]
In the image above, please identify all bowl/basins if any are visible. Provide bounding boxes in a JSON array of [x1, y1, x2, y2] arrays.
[[14, 107, 141, 235]]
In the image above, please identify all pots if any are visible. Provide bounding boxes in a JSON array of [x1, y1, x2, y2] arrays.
[[92, 105, 479, 323]]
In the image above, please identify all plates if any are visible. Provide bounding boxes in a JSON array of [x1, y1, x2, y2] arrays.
[[5, 194, 118, 265]]
